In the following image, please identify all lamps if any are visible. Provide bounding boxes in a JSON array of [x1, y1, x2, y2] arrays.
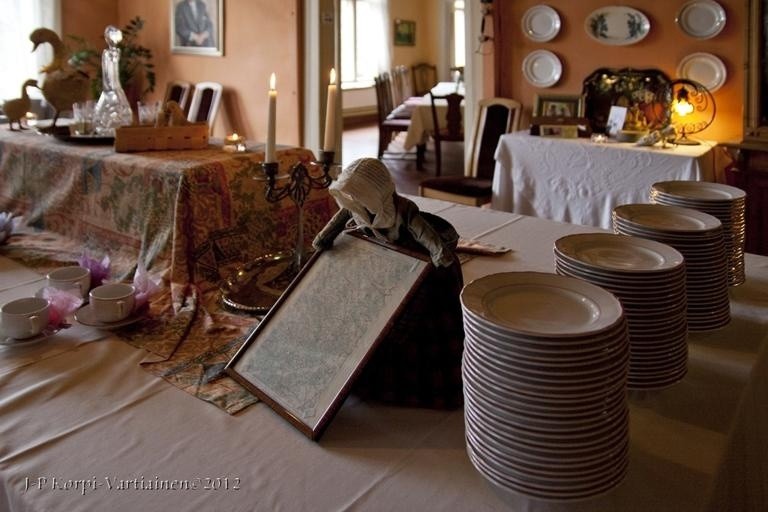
[[651, 78, 717, 145]]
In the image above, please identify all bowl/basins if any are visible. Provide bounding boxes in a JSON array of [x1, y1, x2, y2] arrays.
[[616, 129, 649, 143]]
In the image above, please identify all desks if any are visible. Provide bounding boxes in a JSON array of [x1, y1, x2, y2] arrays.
[[499, 127, 719, 227], [0, 190, 768, 512]]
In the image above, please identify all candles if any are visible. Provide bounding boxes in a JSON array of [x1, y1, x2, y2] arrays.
[[323, 67, 338, 152], [265, 72, 277, 164]]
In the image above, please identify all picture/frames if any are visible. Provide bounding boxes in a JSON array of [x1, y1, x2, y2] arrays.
[[393, 19, 417, 47], [169, 0, 225, 58], [532, 92, 586, 119], [224, 228, 433, 441]]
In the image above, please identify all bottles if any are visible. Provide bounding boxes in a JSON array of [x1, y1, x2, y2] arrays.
[[93, 24, 133, 136]]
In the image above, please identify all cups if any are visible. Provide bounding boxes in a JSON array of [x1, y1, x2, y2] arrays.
[[2, 297, 50, 339], [46, 267, 92, 300], [138, 101, 162, 125], [69, 100, 95, 135], [90, 283, 135, 323]]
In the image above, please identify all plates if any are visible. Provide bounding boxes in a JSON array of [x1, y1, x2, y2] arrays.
[[649, 181, 746, 289], [553, 234, 687, 393], [521, 49, 562, 89], [676, 52, 727, 96], [74, 304, 149, 329], [675, 0, 727, 40], [611, 203, 732, 334], [460, 271, 630, 503], [583, 6, 650, 46], [219, 251, 293, 310], [520, 5, 560, 42], [0, 329, 62, 348], [33, 286, 89, 308]]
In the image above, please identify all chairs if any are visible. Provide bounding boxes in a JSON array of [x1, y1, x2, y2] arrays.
[[415, 96, 523, 209], [374, 61, 463, 177], [162, 79, 224, 137]]
